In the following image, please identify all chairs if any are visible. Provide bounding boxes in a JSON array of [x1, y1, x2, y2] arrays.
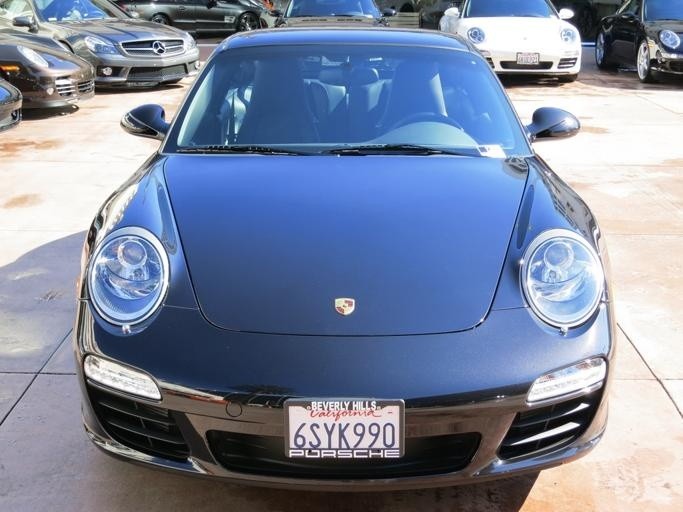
[[234, 51, 480, 149]]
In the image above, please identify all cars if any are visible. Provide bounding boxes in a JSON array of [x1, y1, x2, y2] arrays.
[[75, 26, 613, 495]]
